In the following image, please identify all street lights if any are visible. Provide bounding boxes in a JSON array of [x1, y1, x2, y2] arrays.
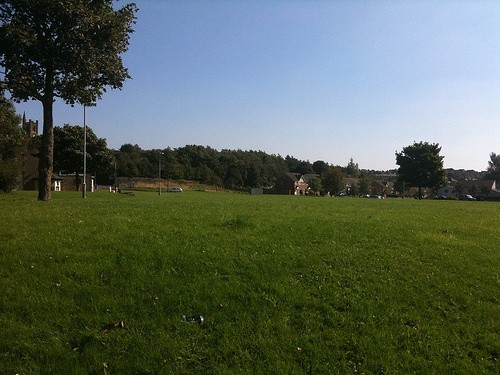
[[158, 152, 164, 196]]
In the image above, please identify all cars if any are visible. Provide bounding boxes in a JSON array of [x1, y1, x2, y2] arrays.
[[459, 194, 477, 201], [169, 187, 183, 192]]
